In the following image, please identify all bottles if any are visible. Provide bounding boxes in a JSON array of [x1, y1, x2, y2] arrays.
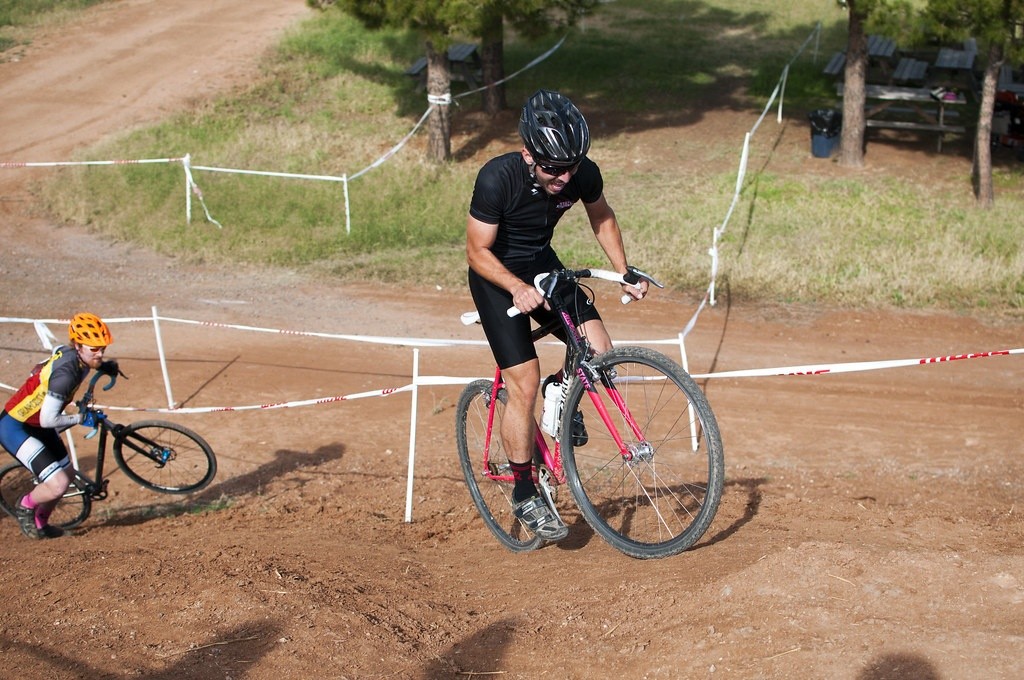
[[540, 378, 562, 437]]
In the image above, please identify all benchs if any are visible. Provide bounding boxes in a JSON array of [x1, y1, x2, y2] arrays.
[[964, 36, 978, 56], [404, 56, 428, 95], [891, 58, 928, 88], [862, 106, 965, 152], [999, 65, 1012, 86], [823, 51, 846, 76]]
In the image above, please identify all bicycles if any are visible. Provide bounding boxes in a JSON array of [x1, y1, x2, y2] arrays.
[[0, 361, 219, 536], [454, 267, 724, 559]]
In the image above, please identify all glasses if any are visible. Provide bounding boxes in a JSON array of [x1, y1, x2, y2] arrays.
[[529, 154, 581, 176], [78, 344, 109, 353]]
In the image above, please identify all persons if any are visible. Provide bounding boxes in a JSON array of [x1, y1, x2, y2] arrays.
[[467, 90, 649, 548], [0, 312, 113, 540]]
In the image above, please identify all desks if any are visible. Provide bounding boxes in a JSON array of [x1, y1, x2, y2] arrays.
[[1003, 83, 1024, 101], [447, 41, 481, 97], [834, 82, 966, 153], [865, 34, 900, 84], [935, 47, 978, 100]]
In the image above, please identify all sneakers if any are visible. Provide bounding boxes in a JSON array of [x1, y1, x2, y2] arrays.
[[14, 493, 43, 542], [510, 488, 568, 542], [542, 375, 589, 447], [37, 526, 74, 539]]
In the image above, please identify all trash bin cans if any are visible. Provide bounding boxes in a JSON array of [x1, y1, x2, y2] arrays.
[[808, 107, 842, 158]]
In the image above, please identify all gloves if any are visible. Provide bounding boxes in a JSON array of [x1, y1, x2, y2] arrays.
[[78, 412, 100, 429], [96, 360, 121, 376]]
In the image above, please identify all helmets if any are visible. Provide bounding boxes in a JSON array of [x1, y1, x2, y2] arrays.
[[515, 88, 592, 163], [68, 312, 115, 349]]
